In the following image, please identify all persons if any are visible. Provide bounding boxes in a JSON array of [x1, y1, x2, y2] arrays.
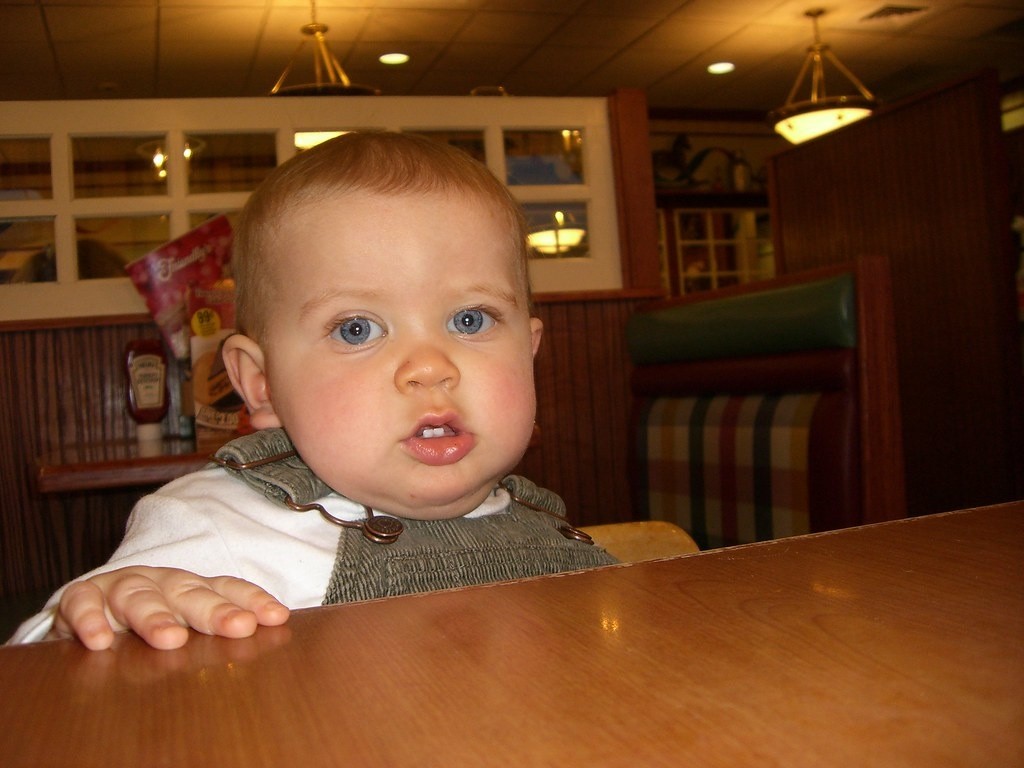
[[4, 133, 623, 651]]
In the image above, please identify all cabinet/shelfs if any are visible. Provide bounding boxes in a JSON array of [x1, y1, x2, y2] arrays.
[[674, 204, 783, 297]]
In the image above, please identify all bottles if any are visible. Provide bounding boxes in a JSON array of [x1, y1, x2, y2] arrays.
[[727, 149, 751, 191], [122, 341, 171, 440]]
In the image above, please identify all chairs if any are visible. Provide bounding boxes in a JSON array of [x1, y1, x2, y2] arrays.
[[616, 253, 909, 555]]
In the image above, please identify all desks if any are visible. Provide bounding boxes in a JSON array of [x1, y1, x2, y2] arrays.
[[0, 500, 1024, 768], [35, 433, 226, 493]]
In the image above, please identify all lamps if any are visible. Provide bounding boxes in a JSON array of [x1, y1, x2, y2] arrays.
[[265, 1, 383, 151], [765, 9, 875, 145], [520, 204, 587, 256]]
